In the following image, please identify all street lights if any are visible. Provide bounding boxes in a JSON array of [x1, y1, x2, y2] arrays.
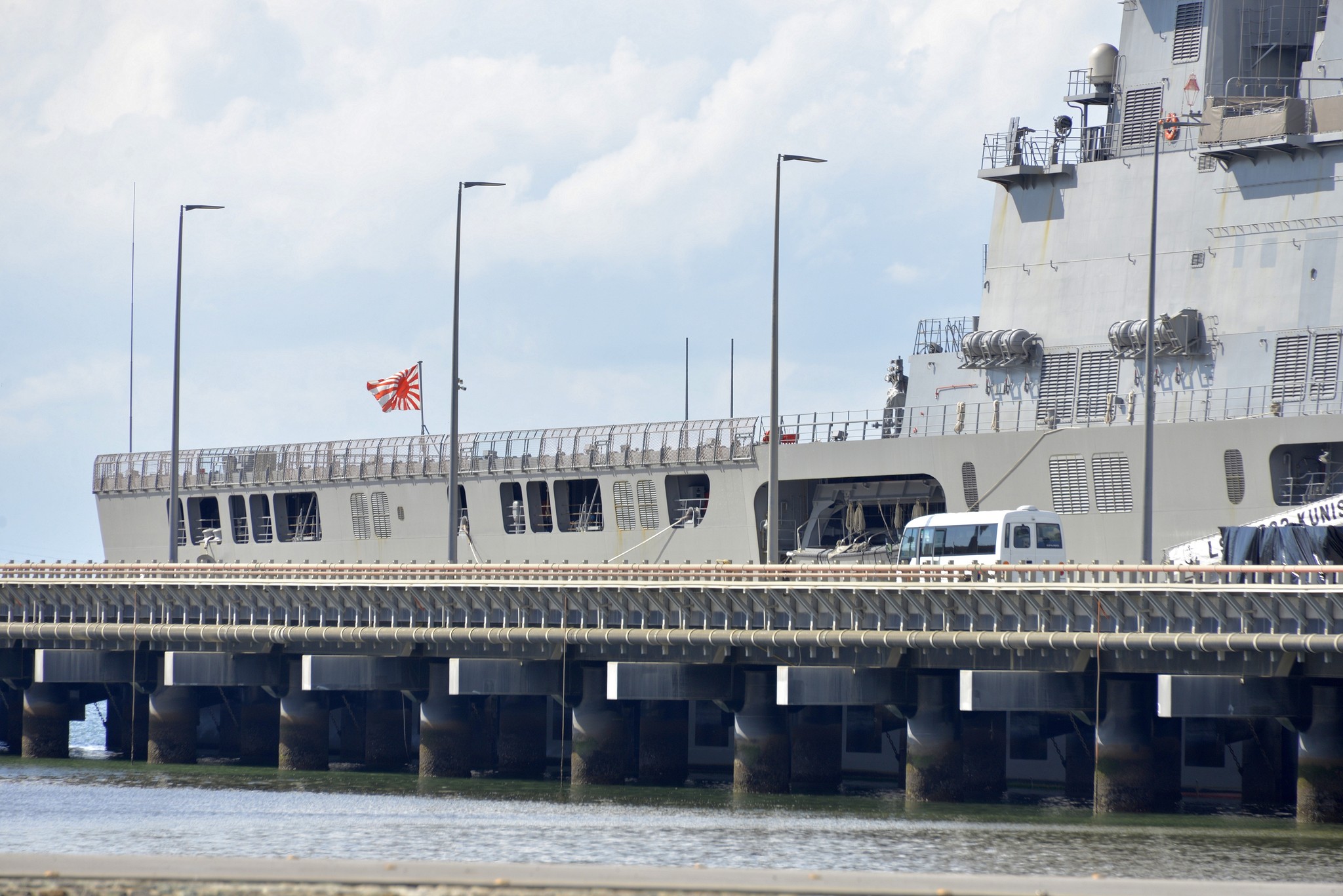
[[1140, 120, 1215, 562], [166, 205, 224, 560], [764, 152, 830, 568], [442, 181, 510, 562]]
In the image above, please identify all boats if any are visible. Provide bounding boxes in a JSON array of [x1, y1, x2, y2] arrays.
[[89, 5, 1343, 575]]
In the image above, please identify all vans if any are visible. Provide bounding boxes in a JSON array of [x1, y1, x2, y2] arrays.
[[885, 505, 1068, 584]]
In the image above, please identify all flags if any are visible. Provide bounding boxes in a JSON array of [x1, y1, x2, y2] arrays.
[[367, 364, 420, 413]]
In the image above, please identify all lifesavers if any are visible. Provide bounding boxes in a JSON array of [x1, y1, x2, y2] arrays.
[[765, 430, 770, 442], [1163, 113, 1178, 141]]
